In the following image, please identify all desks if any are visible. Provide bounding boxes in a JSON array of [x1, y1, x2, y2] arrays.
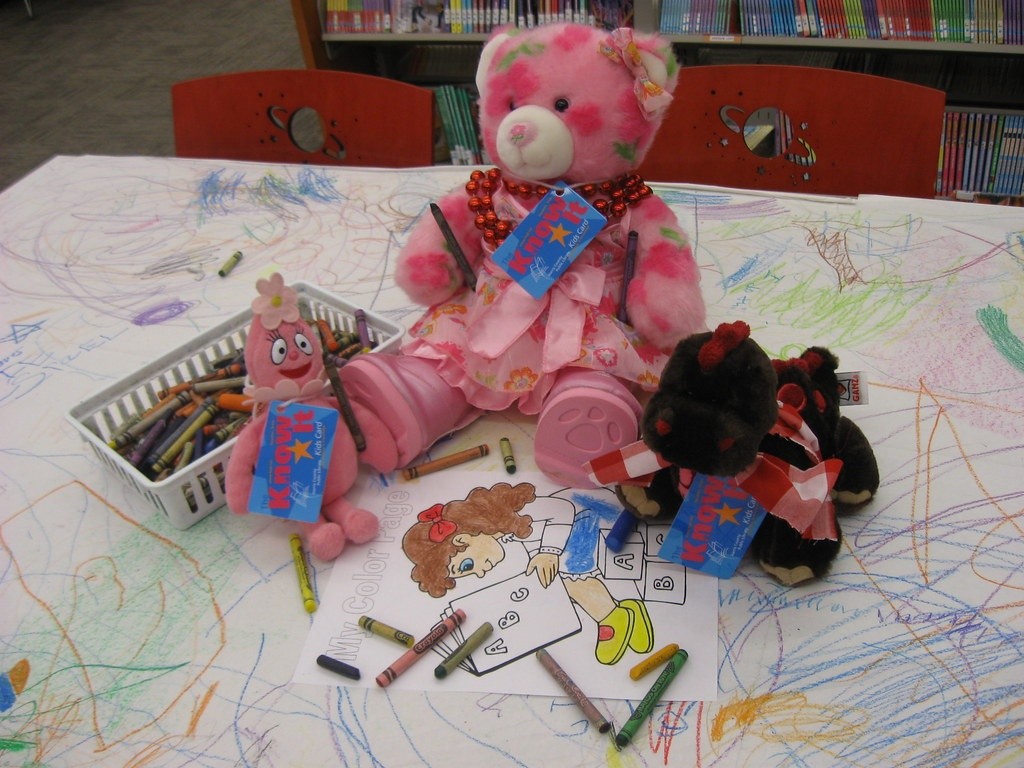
[[0, 155, 1024, 768]]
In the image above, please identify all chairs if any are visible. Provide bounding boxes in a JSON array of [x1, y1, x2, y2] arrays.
[[170, 70, 432, 169], [638, 65, 946, 198]]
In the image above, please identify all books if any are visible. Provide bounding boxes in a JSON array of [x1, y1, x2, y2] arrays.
[[326, 1, 1024, 197]]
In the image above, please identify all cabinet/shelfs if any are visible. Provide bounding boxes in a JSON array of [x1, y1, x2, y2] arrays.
[[279, 0, 1024, 208]]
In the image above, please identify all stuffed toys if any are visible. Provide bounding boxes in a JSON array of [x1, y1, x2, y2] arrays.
[[224, 271, 398, 562], [340, 23, 705, 489], [581, 320, 880, 586]]
